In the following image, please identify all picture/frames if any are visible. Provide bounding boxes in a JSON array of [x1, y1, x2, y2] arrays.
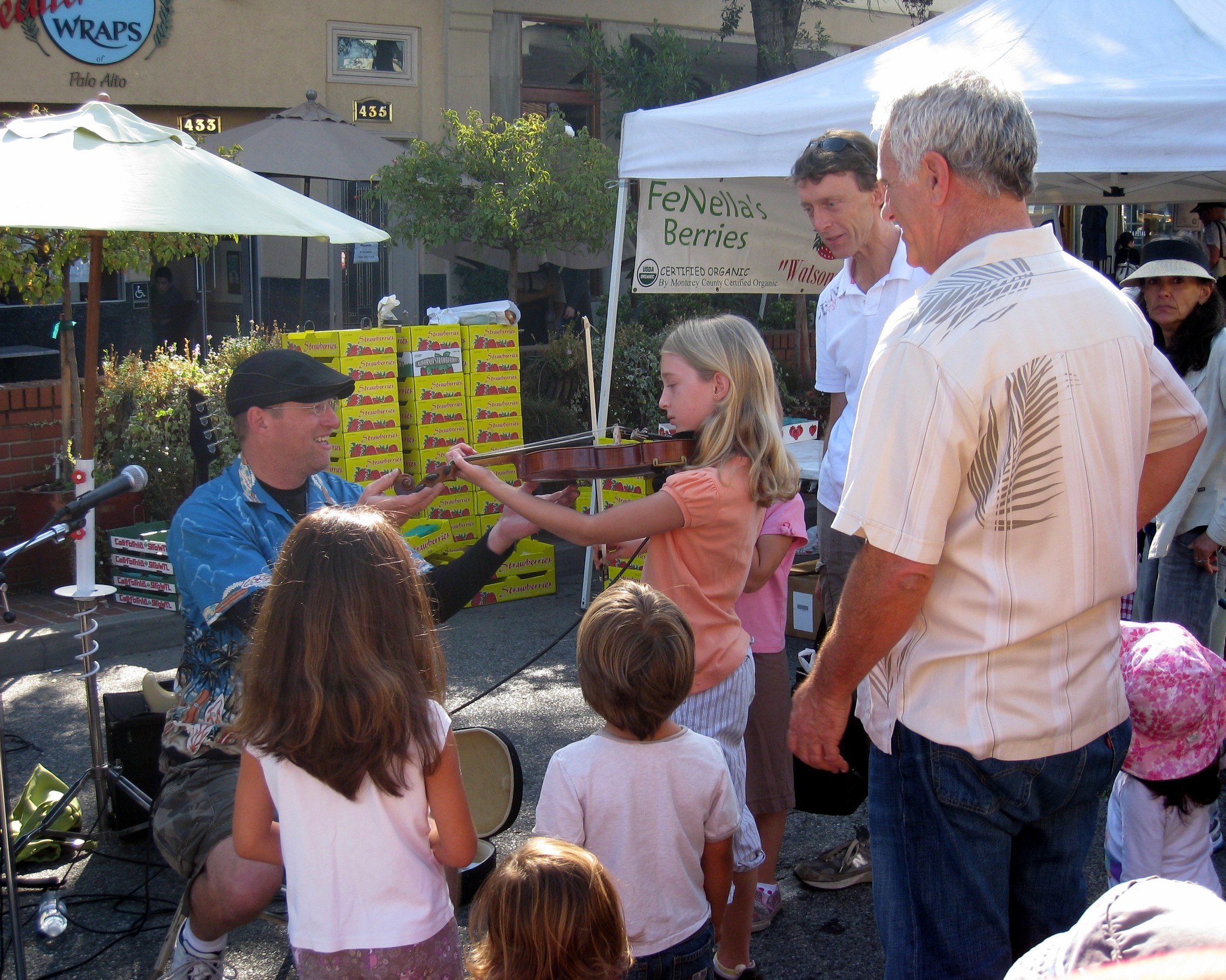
[[227, 250, 242, 295]]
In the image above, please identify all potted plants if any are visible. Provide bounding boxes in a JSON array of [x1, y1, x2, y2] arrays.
[[15, 484, 145, 591]]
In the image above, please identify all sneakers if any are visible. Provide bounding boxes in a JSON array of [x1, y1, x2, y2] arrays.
[[792, 826, 874, 890], [750, 882, 783, 932]]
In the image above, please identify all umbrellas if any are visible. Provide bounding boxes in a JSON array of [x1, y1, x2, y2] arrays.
[[0, 88, 636, 450]]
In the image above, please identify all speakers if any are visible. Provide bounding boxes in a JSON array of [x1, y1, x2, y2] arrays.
[[104, 680, 168, 829]]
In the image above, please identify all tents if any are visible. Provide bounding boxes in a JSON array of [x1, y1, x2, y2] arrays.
[[583, 0, 1225, 608]]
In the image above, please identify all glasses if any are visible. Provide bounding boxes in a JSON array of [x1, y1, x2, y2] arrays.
[[262, 396, 340, 415]]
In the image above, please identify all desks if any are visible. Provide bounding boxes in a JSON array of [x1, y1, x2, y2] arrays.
[[0, 344, 58, 384], [783, 440, 824, 479]]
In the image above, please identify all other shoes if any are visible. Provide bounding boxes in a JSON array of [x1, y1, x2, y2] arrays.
[[155, 917, 238, 980], [713, 950, 763, 980]]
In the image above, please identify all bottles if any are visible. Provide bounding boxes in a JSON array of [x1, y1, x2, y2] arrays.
[[37, 890, 68, 938]]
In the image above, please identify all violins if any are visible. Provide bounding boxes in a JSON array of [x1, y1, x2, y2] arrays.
[[393, 424, 700, 496]]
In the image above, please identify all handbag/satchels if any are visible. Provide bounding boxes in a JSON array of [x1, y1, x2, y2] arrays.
[[792, 613, 869, 815], [14, 764, 98, 862], [1115, 259, 1140, 283], [1211, 257, 1226, 277]]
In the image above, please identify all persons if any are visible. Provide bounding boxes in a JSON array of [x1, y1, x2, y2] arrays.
[[138, 77, 1226, 980]]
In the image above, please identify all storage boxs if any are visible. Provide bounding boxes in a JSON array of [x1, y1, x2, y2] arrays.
[[281, 323, 556, 608], [576, 421, 823, 642], [108, 520, 179, 612]]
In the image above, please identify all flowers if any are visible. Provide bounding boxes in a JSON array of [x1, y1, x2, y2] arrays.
[[32, 440, 112, 492]]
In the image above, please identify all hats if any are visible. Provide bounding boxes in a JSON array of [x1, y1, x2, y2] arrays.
[[1118, 240, 1217, 287], [225, 350, 355, 417], [1189, 203, 1226, 213], [1004, 874, 1226, 980], [1118, 618, 1226, 782], [1147, 218, 1160, 231]]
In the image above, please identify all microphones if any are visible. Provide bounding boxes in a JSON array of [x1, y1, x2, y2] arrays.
[[52, 463, 148, 520]]
